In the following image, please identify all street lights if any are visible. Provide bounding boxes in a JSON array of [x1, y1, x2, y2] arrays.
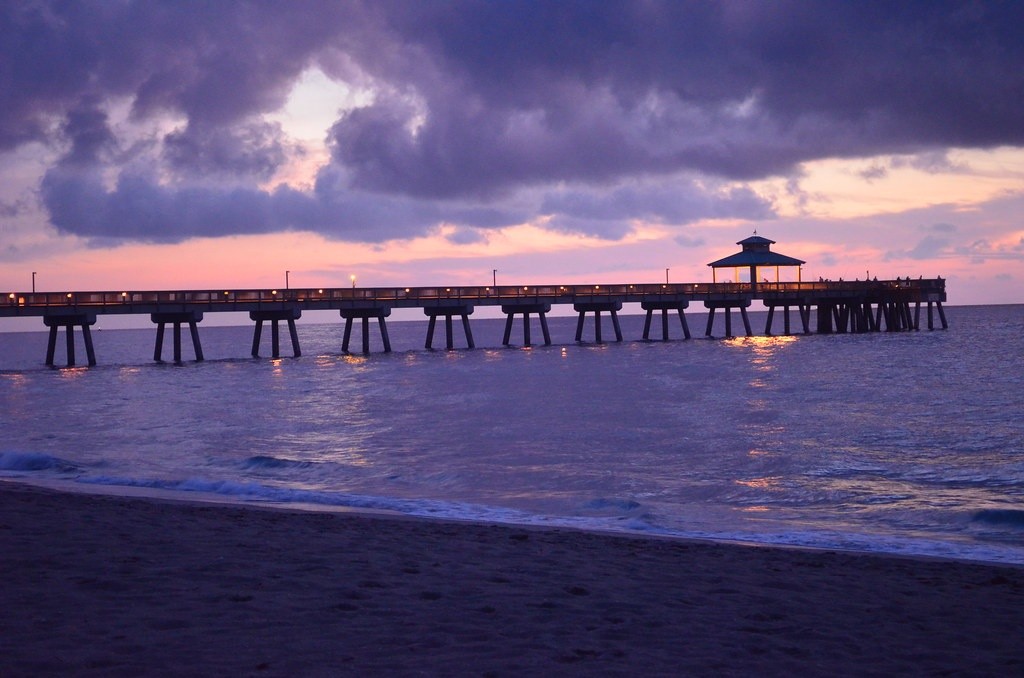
[[31, 271, 38, 293], [493, 269, 498, 287], [286, 270, 290, 290], [665, 268, 670, 284]]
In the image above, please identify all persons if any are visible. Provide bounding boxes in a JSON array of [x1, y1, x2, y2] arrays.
[[819, 275, 941, 281]]
